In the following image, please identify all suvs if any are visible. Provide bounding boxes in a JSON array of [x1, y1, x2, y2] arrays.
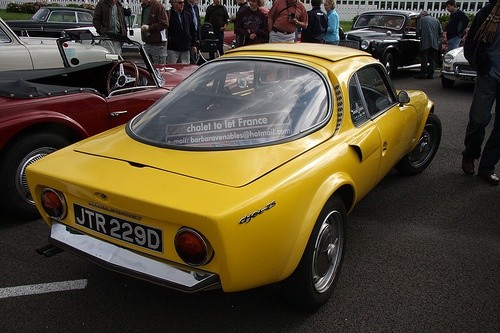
[[339, 9, 421, 78], [4, 6, 97, 38]]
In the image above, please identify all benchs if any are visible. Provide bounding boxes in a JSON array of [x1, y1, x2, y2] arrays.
[[15, 78, 103, 98]]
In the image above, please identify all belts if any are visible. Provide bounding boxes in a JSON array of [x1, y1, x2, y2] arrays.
[[277, 29, 294, 34]]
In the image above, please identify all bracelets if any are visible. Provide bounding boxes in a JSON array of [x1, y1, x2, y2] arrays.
[[295, 21, 298, 26]]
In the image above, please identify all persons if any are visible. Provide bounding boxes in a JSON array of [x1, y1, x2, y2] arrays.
[[414, 11, 443, 79], [461, 0, 500, 185], [92, 0, 132, 38], [140, 0, 340, 64], [445, 0, 470, 52]]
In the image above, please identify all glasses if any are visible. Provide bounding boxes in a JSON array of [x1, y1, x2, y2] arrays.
[[178, 1, 185, 4]]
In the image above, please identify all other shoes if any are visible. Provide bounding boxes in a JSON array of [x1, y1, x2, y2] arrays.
[[427, 74, 433, 79], [414, 74, 427, 79], [462, 155, 476, 175], [480, 171, 500, 184]]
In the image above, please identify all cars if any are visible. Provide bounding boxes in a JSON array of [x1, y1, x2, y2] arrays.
[[25, 42, 442, 311], [0, 14, 253, 219], [440, 46, 477, 90]]
